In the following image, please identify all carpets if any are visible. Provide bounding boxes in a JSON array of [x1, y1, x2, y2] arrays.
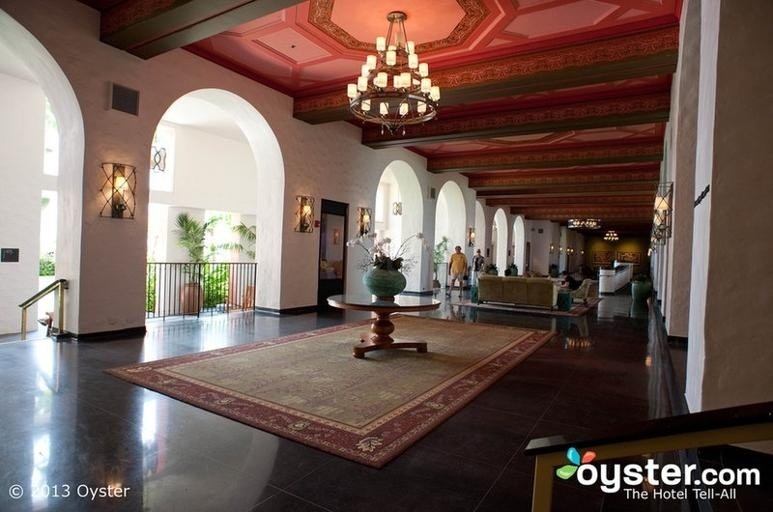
[[102, 314, 559, 468], [447, 298, 604, 317]]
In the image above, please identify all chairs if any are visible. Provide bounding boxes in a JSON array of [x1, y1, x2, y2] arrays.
[[572, 279, 590, 303]]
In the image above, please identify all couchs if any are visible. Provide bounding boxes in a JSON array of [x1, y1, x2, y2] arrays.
[[478, 275, 558, 311], [599, 260, 633, 293]]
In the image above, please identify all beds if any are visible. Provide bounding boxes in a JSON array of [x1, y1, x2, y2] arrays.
[[327, 294, 441, 358]]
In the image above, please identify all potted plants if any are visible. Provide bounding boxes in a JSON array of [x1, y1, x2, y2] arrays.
[[173, 212, 221, 313], [218, 222, 256, 308], [434, 236, 448, 288]]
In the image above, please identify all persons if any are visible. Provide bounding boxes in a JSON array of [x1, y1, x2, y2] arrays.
[[560, 270, 579, 292], [505, 269, 511, 276], [446, 246, 468, 298], [471, 249, 484, 285]]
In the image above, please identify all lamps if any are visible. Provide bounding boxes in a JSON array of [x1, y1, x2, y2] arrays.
[[357, 207, 372, 236], [469, 227, 476, 247], [149, 146, 166, 171], [98, 162, 137, 220], [549, 181, 674, 256], [347, 11, 440, 136], [293, 195, 314, 233], [393, 192, 402, 215]]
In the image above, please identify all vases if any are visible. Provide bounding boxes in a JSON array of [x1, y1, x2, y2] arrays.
[[362, 263, 406, 301], [510, 268, 518, 275], [488, 269, 498, 275], [632, 281, 651, 301]]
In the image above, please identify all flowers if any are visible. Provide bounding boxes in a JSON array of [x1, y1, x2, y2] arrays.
[[346, 233, 424, 273]]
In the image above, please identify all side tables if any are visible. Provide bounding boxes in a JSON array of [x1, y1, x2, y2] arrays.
[[470, 287, 478, 303]]
